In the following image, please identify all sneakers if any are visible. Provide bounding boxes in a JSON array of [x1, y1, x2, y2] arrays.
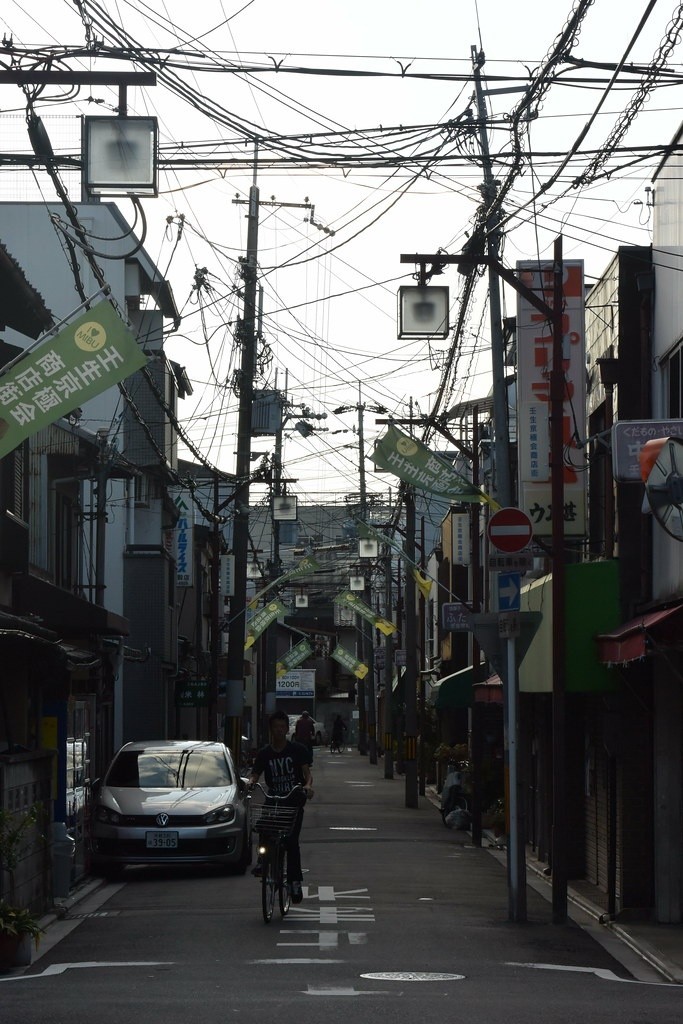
[[290, 881, 303, 904], [251, 863, 264, 877]]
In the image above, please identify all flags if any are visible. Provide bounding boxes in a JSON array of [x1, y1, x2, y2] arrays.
[[380, 533, 433, 601], [250, 554, 321, 611], [331, 643, 369, 678], [245, 599, 286, 650], [334, 589, 397, 636], [275, 640, 312, 681], [367, 423, 497, 509], [0, 298, 150, 461]]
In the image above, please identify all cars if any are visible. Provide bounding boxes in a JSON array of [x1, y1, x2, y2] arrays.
[[286, 714, 325, 745], [90, 740, 253, 877]]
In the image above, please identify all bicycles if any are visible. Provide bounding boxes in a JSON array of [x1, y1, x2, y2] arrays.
[[330, 728, 347, 754], [245, 779, 310, 923]]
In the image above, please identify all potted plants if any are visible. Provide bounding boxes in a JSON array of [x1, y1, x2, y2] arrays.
[[1, 799, 55, 969]]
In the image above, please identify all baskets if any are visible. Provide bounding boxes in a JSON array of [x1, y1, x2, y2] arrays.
[[249, 803, 298, 837]]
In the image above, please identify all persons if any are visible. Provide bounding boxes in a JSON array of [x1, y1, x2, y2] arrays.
[[247, 713, 318, 903], [294, 711, 316, 744], [331, 714, 347, 751]]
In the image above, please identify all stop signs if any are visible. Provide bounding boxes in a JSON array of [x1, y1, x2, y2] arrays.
[[487, 507, 533, 553]]
